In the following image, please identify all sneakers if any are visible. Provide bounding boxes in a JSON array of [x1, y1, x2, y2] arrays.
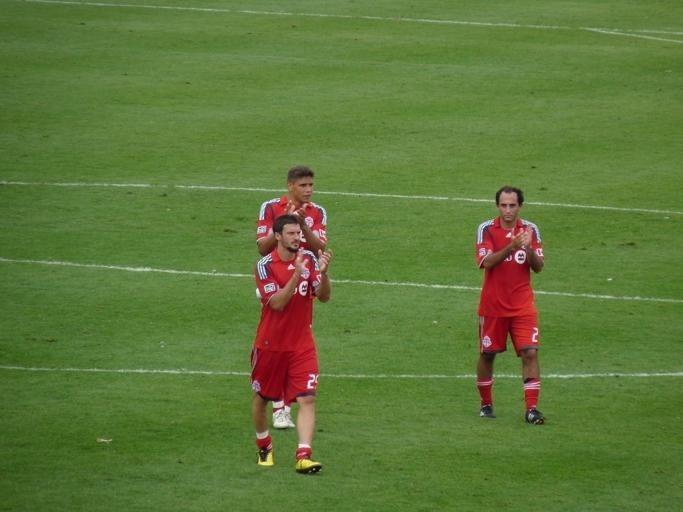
[[273, 409, 295, 428], [527, 410, 545, 423], [296, 458, 321, 471], [479, 405, 495, 418], [258, 449, 274, 465]]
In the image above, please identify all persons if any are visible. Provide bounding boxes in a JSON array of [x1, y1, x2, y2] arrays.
[[252, 164, 329, 431], [245, 213, 324, 478], [472, 183, 549, 426]]
